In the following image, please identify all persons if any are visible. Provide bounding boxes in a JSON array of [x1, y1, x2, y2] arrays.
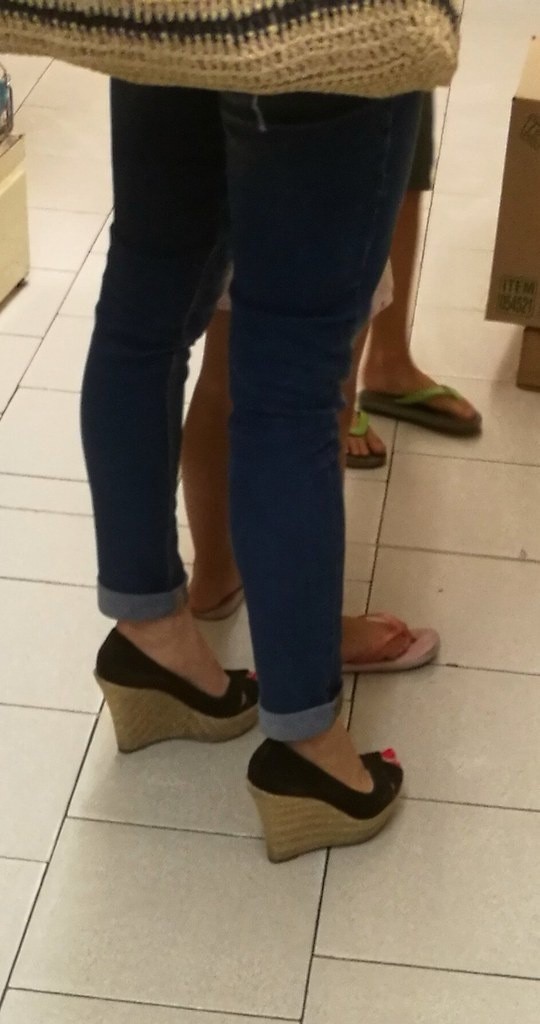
[[344, 90, 482, 470], [80, 0, 404, 864], [179, 259, 442, 673]]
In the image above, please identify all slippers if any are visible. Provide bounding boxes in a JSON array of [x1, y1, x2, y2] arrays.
[[357, 382, 482, 436], [344, 410, 386, 469], [340, 613, 440, 672], [191, 584, 245, 622]]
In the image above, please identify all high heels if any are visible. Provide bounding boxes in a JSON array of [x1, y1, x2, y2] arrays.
[[245, 738, 403, 864], [93, 627, 261, 753]]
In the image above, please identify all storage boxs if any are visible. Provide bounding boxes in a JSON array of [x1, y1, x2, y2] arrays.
[[484, 34, 540, 394]]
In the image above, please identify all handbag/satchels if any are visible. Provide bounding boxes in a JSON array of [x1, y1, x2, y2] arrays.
[[0, 0, 464, 98]]
